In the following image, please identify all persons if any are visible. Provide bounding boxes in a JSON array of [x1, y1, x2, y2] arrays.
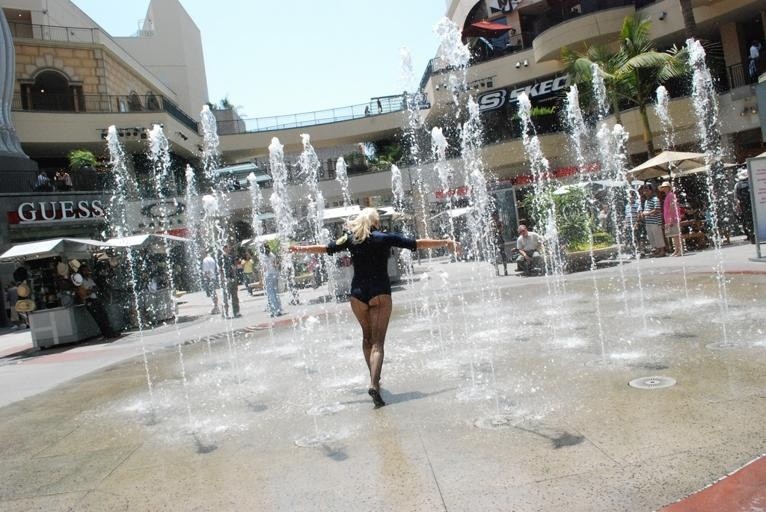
[[287, 207, 462, 408], [31, 172, 52, 192], [596, 168, 757, 258], [364, 105, 372, 117], [376, 98, 382, 114], [51, 168, 73, 191], [749, 38, 765, 77], [200, 242, 325, 319], [0, 252, 157, 338], [748, 53, 758, 83], [515, 223, 549, 275]]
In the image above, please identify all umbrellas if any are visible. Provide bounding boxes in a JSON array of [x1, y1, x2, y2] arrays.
[[461, 17, 514, 56], [629, 149, 722, 181], [661, 162, 740, 180]]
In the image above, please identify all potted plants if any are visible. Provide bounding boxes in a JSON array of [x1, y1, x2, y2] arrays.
[[69, 149, 97, 176]]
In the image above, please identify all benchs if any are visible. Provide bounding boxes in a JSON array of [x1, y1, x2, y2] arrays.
[[248, 271, 315, 296], [680, 217, 730, 244]]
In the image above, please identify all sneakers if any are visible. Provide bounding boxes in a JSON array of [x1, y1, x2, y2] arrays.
[[369, 387, 384, 407], [11, 324, 18, 329], [25, 323, 30, 329]]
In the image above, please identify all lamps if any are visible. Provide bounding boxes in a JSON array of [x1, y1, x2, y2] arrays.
[[515, 59, 529, 69], [659, 11, 666, 20], [740, 107, 757, 117], [436, 83, 447, 91]]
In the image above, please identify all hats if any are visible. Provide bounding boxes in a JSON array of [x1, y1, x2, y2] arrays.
[[57, 263, 68, 275], [17, 282, 31, 298], [15, 300, 36, 313], [69, 260, 81, 272], [13, 266, 27, 282], [658, 181, 672, 192], [71, 273, 83, 286]]
